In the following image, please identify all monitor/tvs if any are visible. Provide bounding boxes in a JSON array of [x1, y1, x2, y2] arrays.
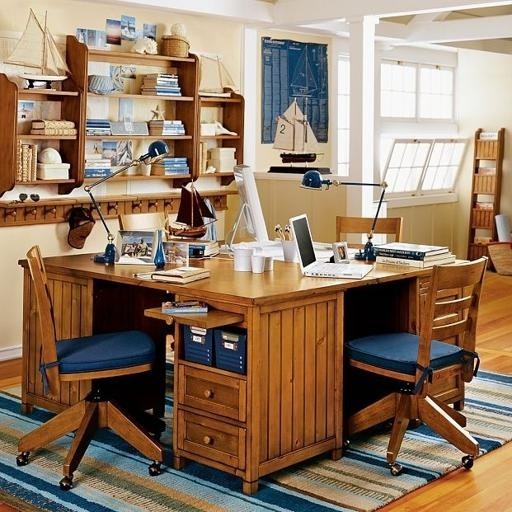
[[225, 164, 269, 253]]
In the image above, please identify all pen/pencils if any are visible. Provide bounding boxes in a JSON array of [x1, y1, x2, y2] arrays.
[[274, 225, 292, 241]]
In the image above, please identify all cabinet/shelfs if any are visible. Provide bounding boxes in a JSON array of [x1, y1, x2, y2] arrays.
[[0, 32, 246, 199], [0, 188, 240, 228]]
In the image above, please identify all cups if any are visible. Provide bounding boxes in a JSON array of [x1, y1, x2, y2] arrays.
[[233, 248, 254, 272], [251, 255, 265, 274], [264, 257, 273, 272]]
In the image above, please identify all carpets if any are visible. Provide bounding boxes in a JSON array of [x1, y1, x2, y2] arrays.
[[0, 369, 512, 512]]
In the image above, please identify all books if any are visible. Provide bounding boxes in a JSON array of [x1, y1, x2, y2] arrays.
[[156, 155, 190, 174], [472, 236, 493, 244], [149, 266, 210, 285], [149, 119, 185, 134], [157, 301, 209, 316], [190, 239, 221, 258], [478, 132, 498, 141], [18, 140, 38, 181], [475, 165, 496, 174], [84, 154, 113, 177], [475, 201, 494, 210], [86, 119, 111, 136], [372, 241, 457, 268], [28, 119, 78, 135], [137, 73, 183, 96]]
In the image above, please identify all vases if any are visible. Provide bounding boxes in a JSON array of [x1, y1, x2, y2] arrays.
[[153, 229, 166, 271]]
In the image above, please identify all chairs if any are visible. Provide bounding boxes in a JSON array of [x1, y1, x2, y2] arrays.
[[343, 255, 489, 477], [335, 215, 403, 250], [16, 244, 166, 491]]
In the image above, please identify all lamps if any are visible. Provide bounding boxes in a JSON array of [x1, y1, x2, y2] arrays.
[[84, 139, 169, 264], [298, 170, 389, 261]]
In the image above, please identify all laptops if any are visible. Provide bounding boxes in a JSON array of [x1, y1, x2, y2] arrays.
[[289, 213, 374, 279]]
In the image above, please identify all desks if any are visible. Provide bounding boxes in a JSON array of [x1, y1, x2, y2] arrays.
[[17, 239, 471, 496]]
